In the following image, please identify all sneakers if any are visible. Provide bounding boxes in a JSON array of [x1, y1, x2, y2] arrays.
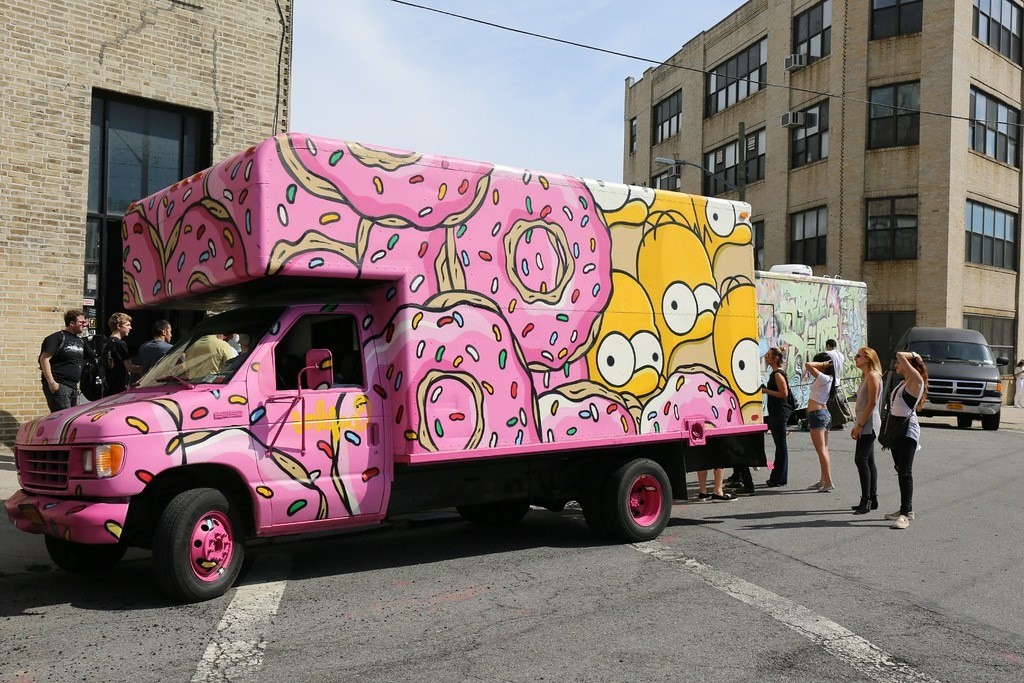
[[699, 491, 712, 501], [712, 491, 737, 502], [893, 515, 910, 528], [885, 509, 915, 520]]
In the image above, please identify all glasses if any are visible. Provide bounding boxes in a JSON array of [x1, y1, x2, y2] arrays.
[[766, 354, 777, 357], [75, 320, 86, 324], [855, 354, 865, 358]]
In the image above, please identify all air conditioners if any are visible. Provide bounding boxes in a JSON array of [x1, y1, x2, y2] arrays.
[[780, 111, 803, 128], [667, 164, 680, 177], [784, 53, 806, 71]]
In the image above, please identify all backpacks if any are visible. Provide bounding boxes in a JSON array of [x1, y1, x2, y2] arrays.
[[80, 339, 118, 400]]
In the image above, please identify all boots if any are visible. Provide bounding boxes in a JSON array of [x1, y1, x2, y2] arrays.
[[855, 497, 872, 514], [852, 495, 878, 510]]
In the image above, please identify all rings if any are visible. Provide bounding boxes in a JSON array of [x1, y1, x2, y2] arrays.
[[852, 435, 856, 438]]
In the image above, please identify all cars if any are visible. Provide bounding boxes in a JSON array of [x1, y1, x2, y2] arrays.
[[882, 328, 1010, 434]]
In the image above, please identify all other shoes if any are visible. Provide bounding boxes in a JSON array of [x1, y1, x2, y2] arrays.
[[766, 480, 784, 487], [1014, 404, 1021, 408], [733, 486, 755, 495], [728, 474, 743, 483], [725, 483, 742, 488], [808, 482, 821, 490], [819, 483, 835, 491]]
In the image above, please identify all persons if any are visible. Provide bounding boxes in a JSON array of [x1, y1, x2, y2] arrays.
[[804, 352, 836, 491], [182, 331, 235, 379], [824, 338, 846, 430], [39, 309, 97, 413], [760, 347, 793, 488], [697, 466, 756, 502], [100, 312, 140, 397], [1014, 358, 1024, 408], [880, 352, 928, 529], [139, 319, 175, 373], [850, 347, 883, 515]]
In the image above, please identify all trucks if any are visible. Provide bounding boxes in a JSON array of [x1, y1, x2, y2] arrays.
[[0, 132, 781, 607], [748, 263, 868, 425]]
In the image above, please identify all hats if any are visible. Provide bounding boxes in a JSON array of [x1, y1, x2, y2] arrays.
[[1020, 359, 1024, 363]]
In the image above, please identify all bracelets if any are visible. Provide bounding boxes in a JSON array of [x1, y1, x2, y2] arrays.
[[857, 423, 863, 427], [911, 352, 917, 359]]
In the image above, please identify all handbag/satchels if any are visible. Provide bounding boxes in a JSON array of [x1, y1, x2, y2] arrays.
[[878, 418, 909, 449], [789, 389, 799, 411], [828, 390, 853, 426]]
